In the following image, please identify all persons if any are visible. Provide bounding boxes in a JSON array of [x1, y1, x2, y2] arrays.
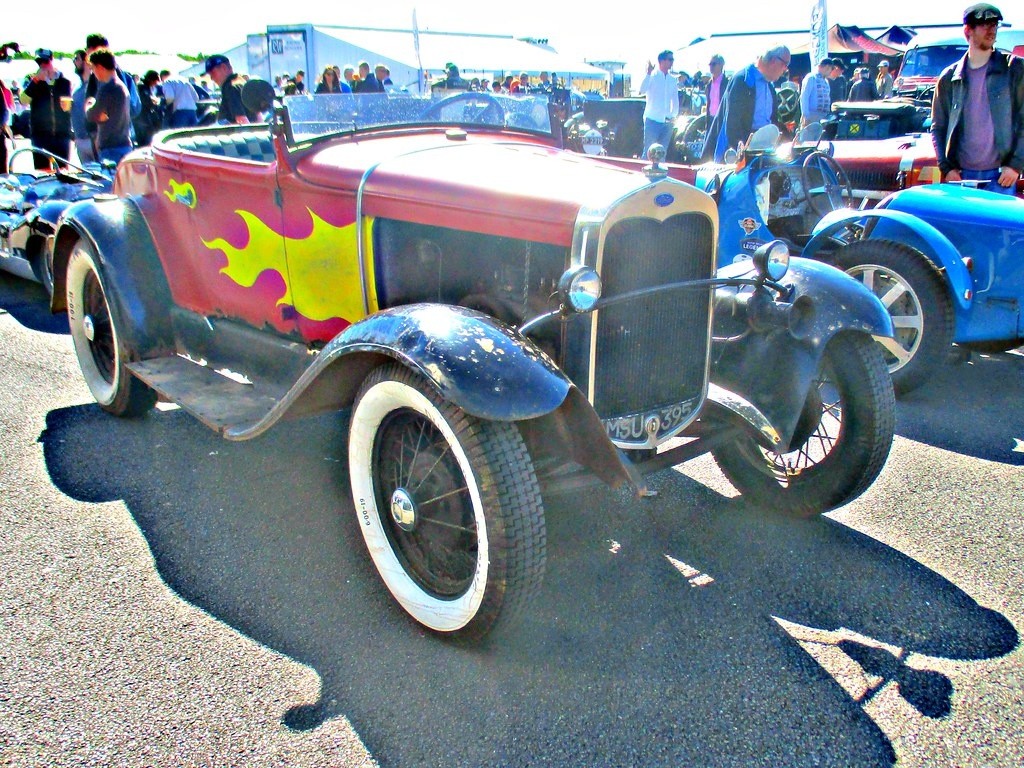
[[931, 3, 1024, 200], [700, 44, 791, 163], [430, 61, 550, 94], [0, 33, 393, 175], [705, 54, 728, 140], [640, 49, 680, 163], [799, 59, 893, 127]]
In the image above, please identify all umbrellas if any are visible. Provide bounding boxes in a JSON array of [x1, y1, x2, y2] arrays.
[[790, 23, 919, 70]]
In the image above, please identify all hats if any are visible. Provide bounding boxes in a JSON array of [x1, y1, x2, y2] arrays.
[[963, 3, 1003, 27], [854, 69, 860, 74], [860, 67, 871, 74], [820, 58, 834, 66], [877, 60, 889, 67], [34, 48, 53, 60], [199, 55, 230, 77], [833, 58, 847, 70]]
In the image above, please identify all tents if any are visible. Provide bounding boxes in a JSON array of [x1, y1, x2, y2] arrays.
[[180, 24, 611, 98]]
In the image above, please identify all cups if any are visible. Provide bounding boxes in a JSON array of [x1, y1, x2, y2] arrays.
[[60, 97, 73, 112], [702, 106, 707, 113]]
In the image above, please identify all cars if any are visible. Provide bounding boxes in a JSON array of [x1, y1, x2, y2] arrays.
[[623, 27, 1024, 398], [0, 89, 897, 651]]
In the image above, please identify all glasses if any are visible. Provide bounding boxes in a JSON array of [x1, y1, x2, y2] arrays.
[[36, 58, 50, 65], [974, 22, 1002, 31], [663, 57, 674, 62], [776, 57, 789, 69], [708, 62, 719, 67]]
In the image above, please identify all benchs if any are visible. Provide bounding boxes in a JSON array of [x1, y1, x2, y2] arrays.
[[165, 130, 317, 163]]
